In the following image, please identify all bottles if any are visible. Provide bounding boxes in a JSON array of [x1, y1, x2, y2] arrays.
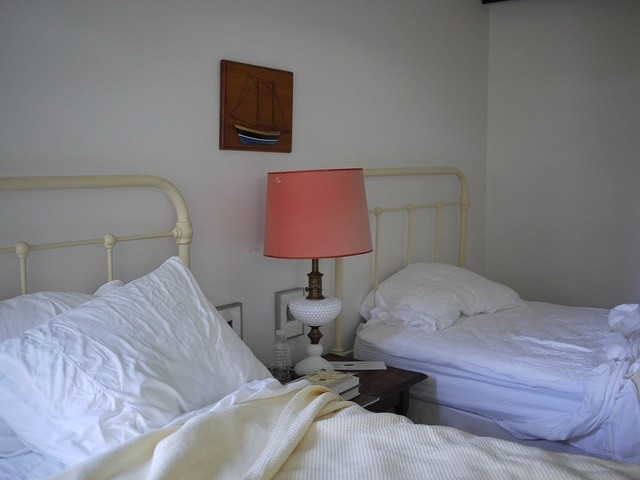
[[272, 330, 291, 385]]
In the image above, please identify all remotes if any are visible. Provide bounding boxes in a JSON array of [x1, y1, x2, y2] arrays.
[[328, 361, 387, 371]]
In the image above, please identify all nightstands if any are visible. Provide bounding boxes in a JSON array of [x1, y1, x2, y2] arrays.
[[267, 352, 429, 417]]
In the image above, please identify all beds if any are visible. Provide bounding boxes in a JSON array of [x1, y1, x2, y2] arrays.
[[329, 167, 639, 464], [2, 174, 640, 480]]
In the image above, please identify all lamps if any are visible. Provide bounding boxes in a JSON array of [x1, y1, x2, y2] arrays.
[[260, 166, 374, 377]]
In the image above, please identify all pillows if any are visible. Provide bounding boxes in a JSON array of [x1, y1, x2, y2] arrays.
[[0, 281, 124, 341], [359, 260, 522, 333], [1, 256, 274, 468]]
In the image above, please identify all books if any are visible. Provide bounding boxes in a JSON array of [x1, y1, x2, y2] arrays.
[[289, 368, 360, 395], [349, 394, 381, 407], [338, 384, 362, 401]]
